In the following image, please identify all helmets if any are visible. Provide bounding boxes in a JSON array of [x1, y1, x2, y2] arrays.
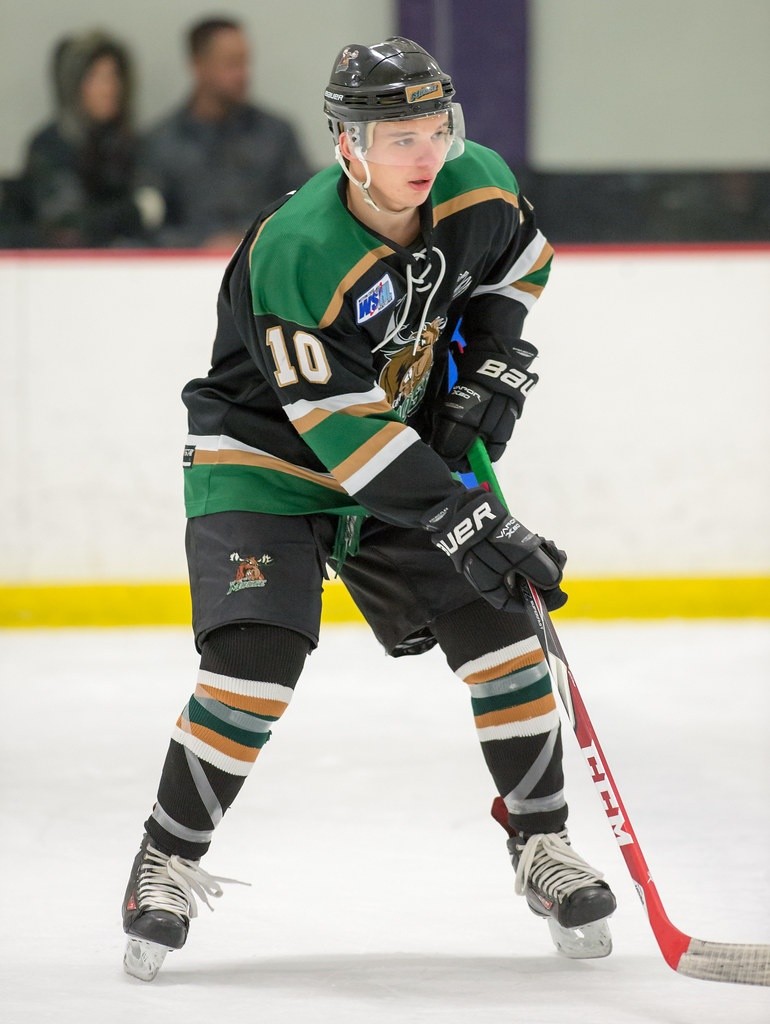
[[323, 36, 457, 140]]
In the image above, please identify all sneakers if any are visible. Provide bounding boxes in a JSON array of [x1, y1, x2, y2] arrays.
[[501, 831, 620, 959], [121, 838, 212, 980]]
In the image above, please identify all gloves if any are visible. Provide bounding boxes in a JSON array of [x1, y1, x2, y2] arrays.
[[418, 478, 568, 619], [426, 332, 541, 473]]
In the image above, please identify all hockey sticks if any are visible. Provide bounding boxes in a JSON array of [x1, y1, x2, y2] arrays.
[[466, 435, 770, 988]]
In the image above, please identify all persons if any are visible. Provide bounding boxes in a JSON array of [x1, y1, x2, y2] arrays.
[[24, 14, 315, 248], [122, 35, 619, 983]]
